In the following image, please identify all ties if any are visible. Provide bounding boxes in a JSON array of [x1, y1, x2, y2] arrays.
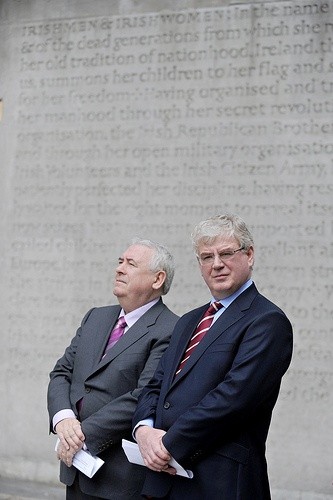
[[105, 316, 127, 351], [176, 302, 224, 375]]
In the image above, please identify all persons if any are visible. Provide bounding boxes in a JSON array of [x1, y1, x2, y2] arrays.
[[47, 237, 181, 500], [131, 213, 294, 500]]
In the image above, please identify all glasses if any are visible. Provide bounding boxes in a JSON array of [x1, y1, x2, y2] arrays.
[[198, 247, 244, 263]]
